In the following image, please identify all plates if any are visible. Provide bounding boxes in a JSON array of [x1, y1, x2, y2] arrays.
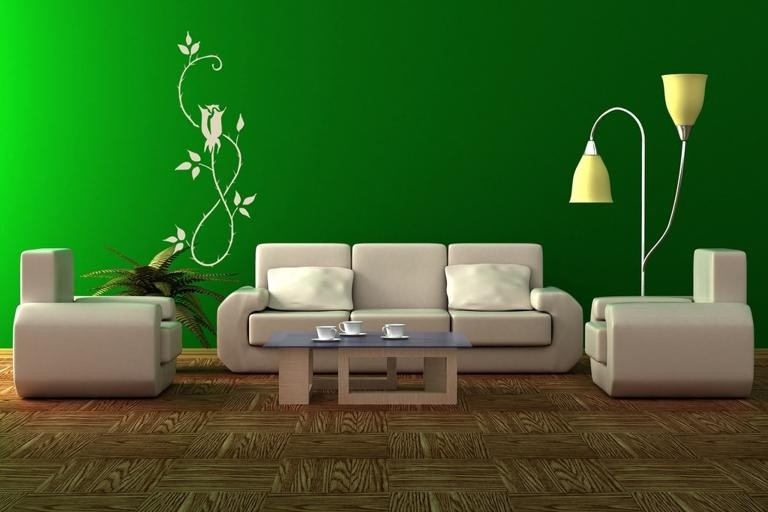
[[311, 331, 367, 342], [377, 337, 411, 341]]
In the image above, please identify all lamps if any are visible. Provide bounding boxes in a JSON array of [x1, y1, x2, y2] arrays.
[[567, 73, 710, 296]]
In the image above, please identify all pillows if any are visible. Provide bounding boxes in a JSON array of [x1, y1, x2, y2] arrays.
[[265, 266, 355, 311], [443, 263, 534, 311]]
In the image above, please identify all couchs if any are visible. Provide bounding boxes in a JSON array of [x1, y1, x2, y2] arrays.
[[214, 241, 586, 375]]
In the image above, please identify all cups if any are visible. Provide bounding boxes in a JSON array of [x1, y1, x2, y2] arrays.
[[340, 320, 363, 334], [316, 323, 338, 338], [381, 324, 406, 338]]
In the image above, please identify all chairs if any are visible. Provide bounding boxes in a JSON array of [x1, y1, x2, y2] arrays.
[[12, 246, 183, 400], [582, 246, 756, 401]]
